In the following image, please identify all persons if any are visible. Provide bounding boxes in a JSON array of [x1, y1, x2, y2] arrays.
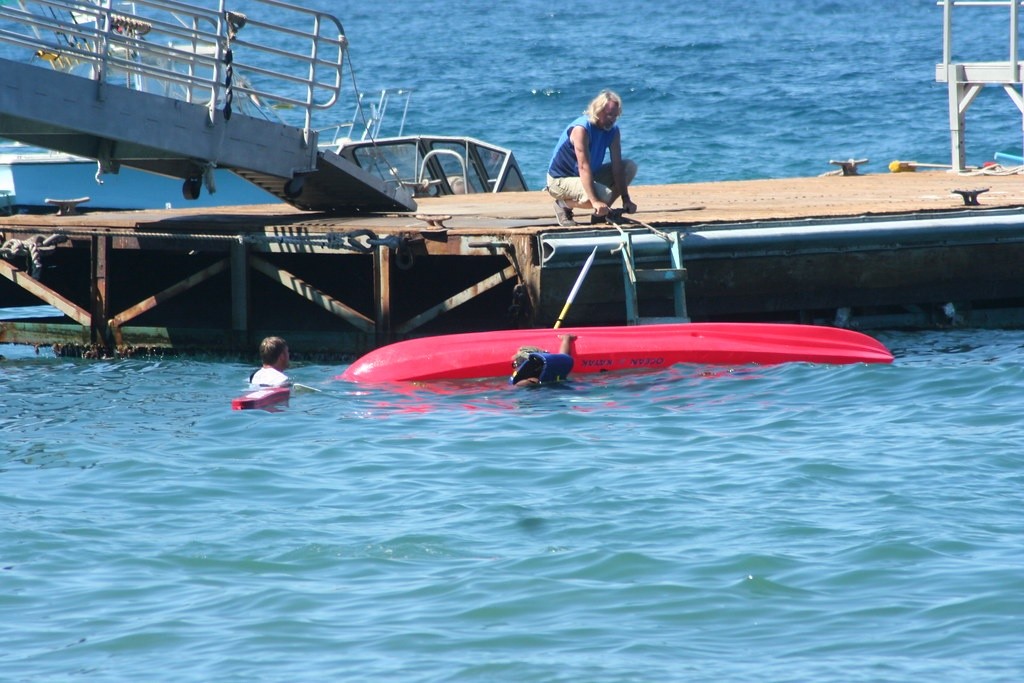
[[546, 91, 637, 227], [508, 334, 576, 386], [248, 336, 293, 388]]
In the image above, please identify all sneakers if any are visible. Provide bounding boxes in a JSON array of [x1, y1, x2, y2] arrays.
[[590, 213, 618, 224], [553, 198, 578, 227]]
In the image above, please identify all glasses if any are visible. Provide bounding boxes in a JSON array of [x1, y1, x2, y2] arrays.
[[512, 362, 521, 369]]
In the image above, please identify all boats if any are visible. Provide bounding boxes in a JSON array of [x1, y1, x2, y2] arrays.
[[333, 320, 895, 383], [1, 133, 529, 210]]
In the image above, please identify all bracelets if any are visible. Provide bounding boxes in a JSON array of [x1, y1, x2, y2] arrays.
[[622, 195, 630, 202]]
